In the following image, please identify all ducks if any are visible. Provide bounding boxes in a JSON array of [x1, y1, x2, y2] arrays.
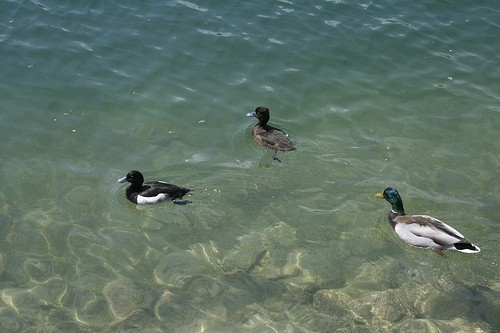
[[117, 170, 195, 206], [374, 186, 482, 259], [246, 106, 296, 164]]
